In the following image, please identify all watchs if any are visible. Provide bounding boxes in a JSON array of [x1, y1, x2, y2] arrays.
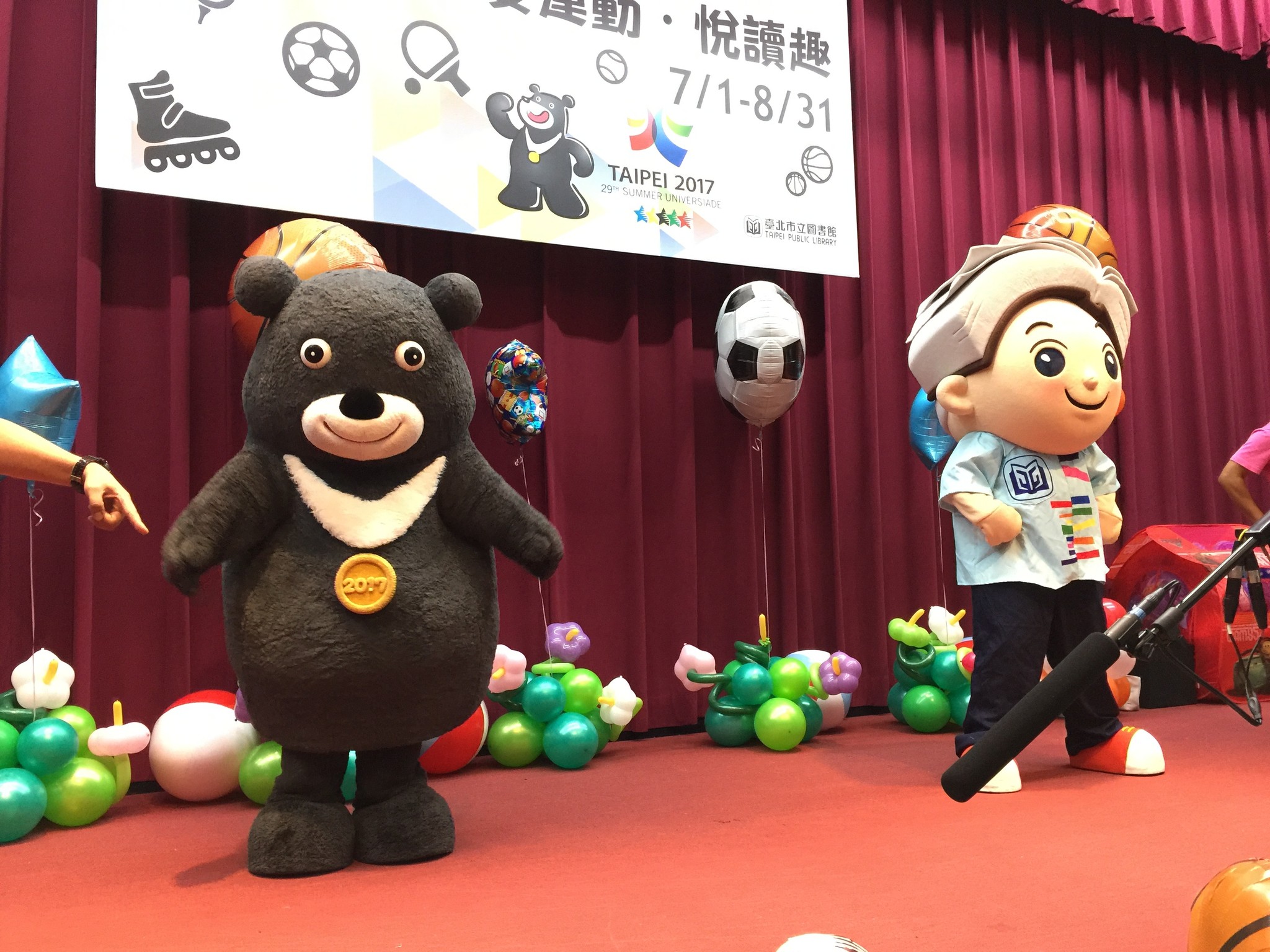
[[70, 454, 110, 493]]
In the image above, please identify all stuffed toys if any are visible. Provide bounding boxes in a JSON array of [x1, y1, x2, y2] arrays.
[[160, 254, 565, 880]]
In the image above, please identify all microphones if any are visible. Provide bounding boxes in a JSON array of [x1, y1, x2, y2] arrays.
[[940, 587, 1168, 804]]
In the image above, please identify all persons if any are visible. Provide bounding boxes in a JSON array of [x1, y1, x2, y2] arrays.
[[902, 239, 1166, 793], [2, 597, 1168, 852], [0, 416, 151, 536], [1218, 421, 1270, 524]]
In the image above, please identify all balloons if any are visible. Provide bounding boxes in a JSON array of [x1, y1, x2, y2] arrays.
[[1006, 201, 1118, 277], [906, 387, 958, 471], [485, 339, 548, 443], [715, 280, 806, 427], [0, 334, 83, 495]]
